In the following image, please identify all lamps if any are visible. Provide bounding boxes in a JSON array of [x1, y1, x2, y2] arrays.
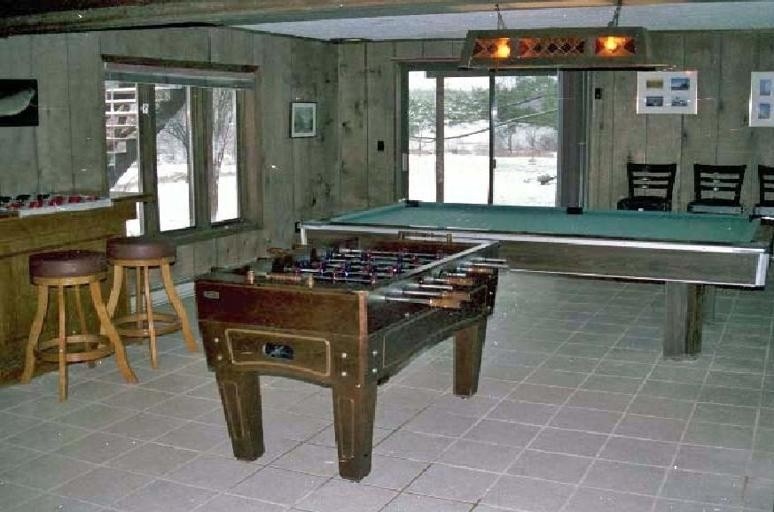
[[458, 7, 654, 72]]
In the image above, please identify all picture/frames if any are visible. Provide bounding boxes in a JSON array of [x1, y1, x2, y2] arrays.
[[290, 102, 317, 140]]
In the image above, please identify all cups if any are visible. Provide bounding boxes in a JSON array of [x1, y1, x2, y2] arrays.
[[68, 196, 81, 204], [48, 196, 63, 207], [30, 201, 43, 209]]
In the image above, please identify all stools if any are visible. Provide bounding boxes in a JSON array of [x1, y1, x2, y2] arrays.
[[21, 250, 139, 400], [98, 237, 198, 370]]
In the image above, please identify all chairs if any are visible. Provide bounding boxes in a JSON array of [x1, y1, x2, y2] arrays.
[[687, 165, 745, 215], [617, 164, 676, 211], [754, 166, 774, 218]]
